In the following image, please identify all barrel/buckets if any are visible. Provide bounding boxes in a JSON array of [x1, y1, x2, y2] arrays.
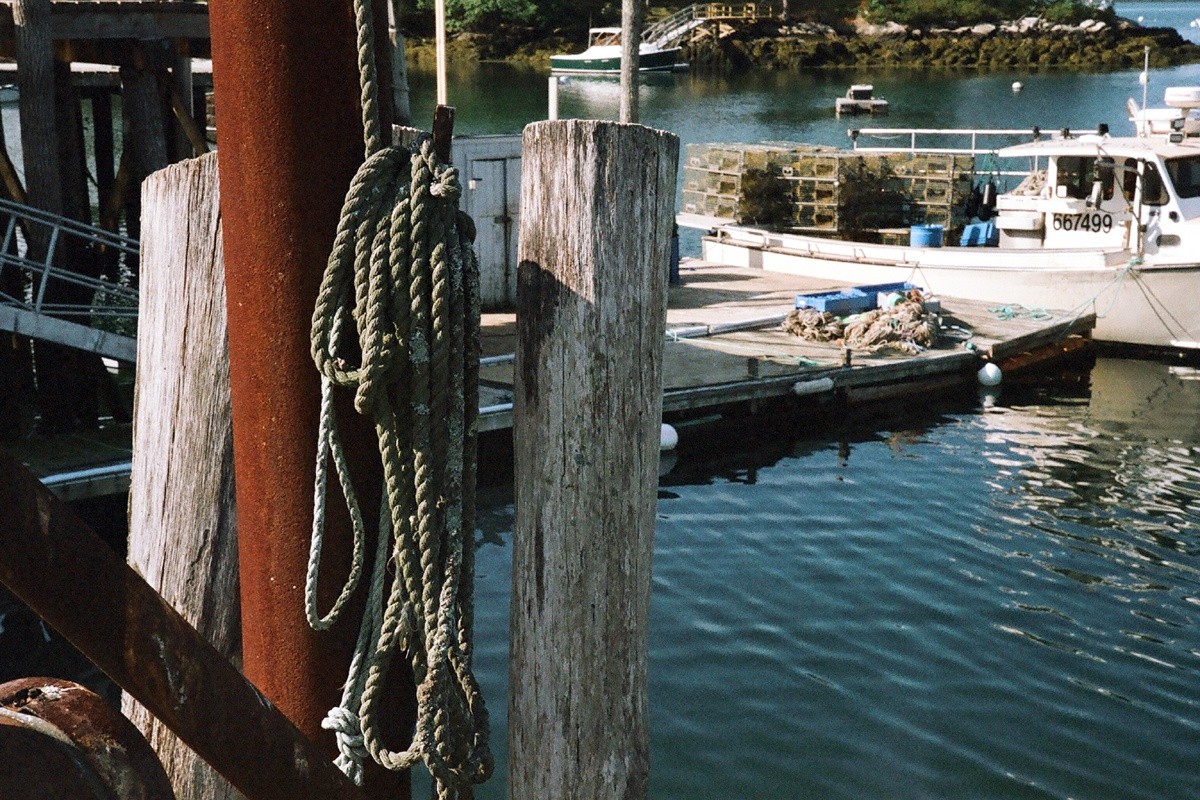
[[910, 224, 944, 248]]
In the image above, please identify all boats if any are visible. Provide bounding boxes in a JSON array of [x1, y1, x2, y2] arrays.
[[663, 36, 1200, 351], [548, 27, 689, 76]]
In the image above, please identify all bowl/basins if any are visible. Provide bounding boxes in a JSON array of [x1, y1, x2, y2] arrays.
[[0, 675, 176, 800]]
